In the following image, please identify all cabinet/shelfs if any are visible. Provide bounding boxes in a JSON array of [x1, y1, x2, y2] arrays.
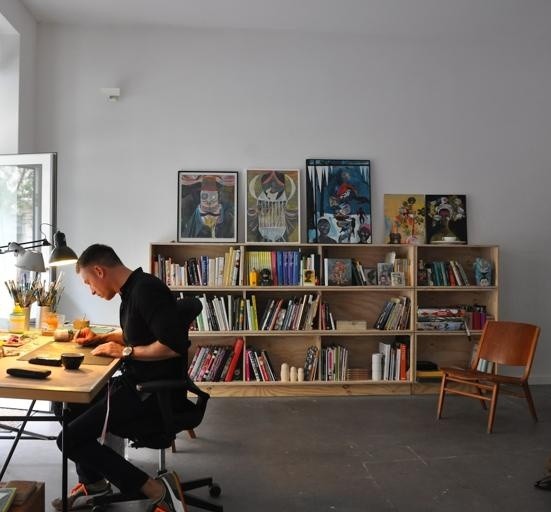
[[412, 243, 500, 398], [148, 243, 413, 397]]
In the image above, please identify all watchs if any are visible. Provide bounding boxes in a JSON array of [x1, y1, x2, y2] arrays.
[[122, 344, 134, 363]]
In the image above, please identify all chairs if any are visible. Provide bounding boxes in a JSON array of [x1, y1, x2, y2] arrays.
[[435, 319, 541, 433], [84, 297, 225, 512]]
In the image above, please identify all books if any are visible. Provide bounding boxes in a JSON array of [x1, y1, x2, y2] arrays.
[[418, 258, 470, 286], [153, 247, 411, 382], [469, 338, 494, 375]]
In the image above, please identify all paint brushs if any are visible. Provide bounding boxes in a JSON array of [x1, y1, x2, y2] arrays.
[[4, 271, 65, 312]]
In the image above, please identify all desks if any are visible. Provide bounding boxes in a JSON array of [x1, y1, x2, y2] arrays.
[[0, 321, 124, 512]]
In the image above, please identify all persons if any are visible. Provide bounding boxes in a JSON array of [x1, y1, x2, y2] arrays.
[[50, 244, 189, 512], [182, 176, 234, 239]]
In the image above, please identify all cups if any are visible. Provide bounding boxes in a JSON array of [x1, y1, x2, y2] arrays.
[[443, 236, 457, 241], [20, 307, 30, 332], [41, 311, 65, 336], [62, 353, 85, 370], [36, 306, 50, 329], [53, 328, 69, 341], [73, 319, 89, 331]]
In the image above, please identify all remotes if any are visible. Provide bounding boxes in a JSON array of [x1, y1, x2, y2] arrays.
[[7, 368, 51, 378], [29, 357, 62, 366]]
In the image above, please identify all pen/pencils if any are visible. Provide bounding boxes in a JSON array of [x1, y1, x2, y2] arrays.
[[75, 328, 82, 344]]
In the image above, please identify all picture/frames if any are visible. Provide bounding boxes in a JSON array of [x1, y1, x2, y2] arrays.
[[243, 168, 300, 244], [305, 159, 373, 245], [176, 170, 239, 243]]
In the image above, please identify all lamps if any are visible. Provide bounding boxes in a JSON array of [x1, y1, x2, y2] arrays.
[[0, 241, 45, 274], [0, 231, 79, 268]]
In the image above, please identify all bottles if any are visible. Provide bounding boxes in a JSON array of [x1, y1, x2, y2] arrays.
[[9, 303, 26, 334], [250, 266, 259, 286], [372, 353, 384, 380], [280, 363, 305, 381]]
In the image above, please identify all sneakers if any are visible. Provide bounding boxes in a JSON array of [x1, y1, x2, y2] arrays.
[[50, 480, 113, 512], [151, 471, 186, 512]]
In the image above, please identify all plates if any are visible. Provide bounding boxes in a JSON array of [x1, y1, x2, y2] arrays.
[[433, 240, 467, 244]]
